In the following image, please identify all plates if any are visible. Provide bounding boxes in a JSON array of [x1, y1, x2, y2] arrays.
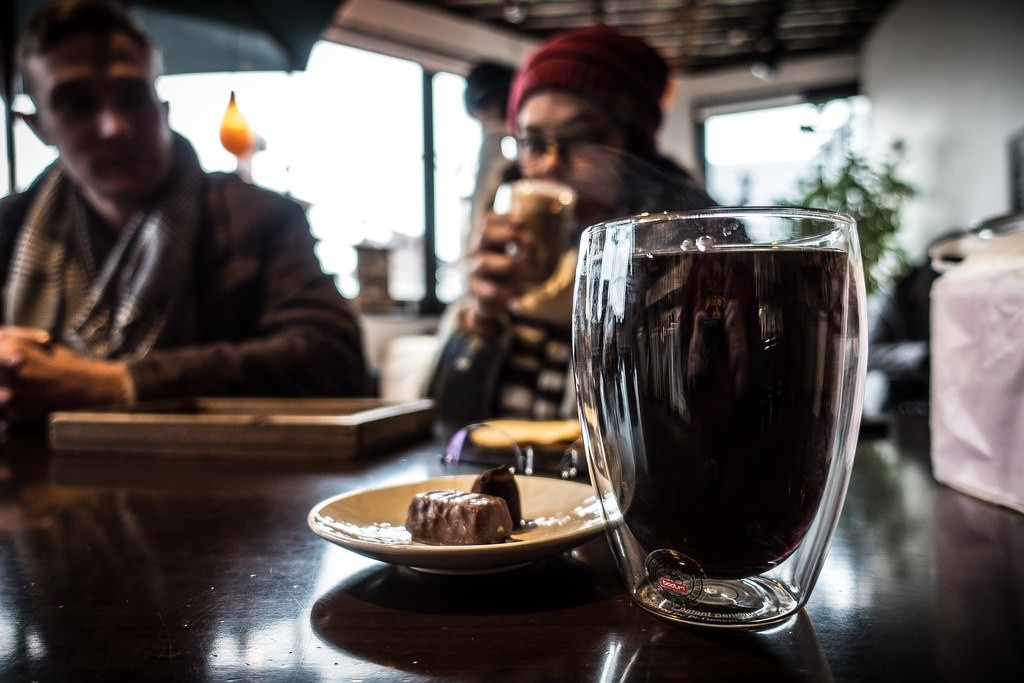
[[307, 474, 625, 575], [469, 418, 581, 456]]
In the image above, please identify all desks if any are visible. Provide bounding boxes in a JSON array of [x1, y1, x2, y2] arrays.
[[0, 418, 1024, 683]]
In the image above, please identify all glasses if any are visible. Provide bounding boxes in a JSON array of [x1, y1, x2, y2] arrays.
[[506, 126, 632, 169]]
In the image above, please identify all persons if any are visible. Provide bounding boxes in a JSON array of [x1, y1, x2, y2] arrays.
[[0, 0, 365, 409], [421, 24, 750, 426], [463, 61, 518, 221]]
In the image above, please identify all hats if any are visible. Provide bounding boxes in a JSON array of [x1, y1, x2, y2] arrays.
[[506, 25, 669, 143]]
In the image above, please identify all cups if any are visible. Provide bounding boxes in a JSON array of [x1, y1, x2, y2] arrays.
[[494, 180, 576, 310], [572, 206, 869, 628]]
